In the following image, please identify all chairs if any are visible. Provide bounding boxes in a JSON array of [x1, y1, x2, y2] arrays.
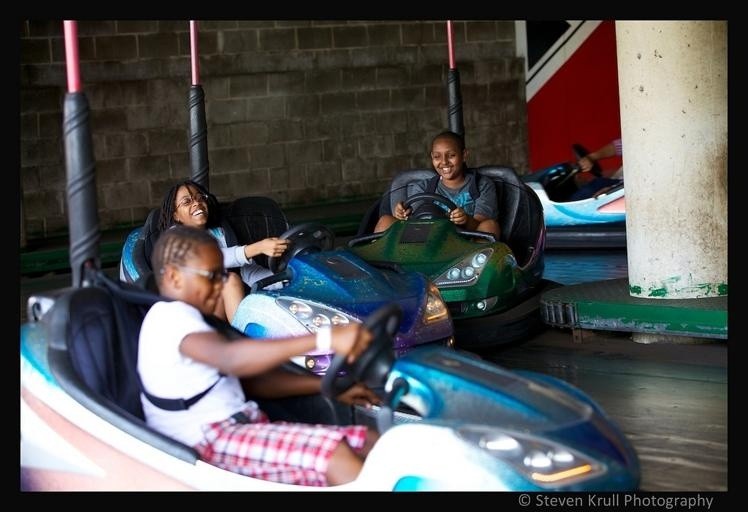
[[142, 195, 289, 270], [64, 280, 157, 420], [389, 166, 531, 252]]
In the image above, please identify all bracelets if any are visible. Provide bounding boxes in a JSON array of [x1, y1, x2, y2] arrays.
[[315, 324, 331, 352]]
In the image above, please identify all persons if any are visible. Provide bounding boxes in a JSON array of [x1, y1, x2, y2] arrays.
[[116, 194, 457, 352], [333, 163, 547, 333], [373, 130, 500, 244], [156, 179, 292, 296], [512, 142, 628, 249], [133, 223, 379, 484], [577, 138, 623, 174], [21, 273, 644, 494]]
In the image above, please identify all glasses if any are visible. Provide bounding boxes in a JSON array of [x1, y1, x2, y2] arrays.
[[160, 263, 230, 284], [175, 192, 210, 212]]
